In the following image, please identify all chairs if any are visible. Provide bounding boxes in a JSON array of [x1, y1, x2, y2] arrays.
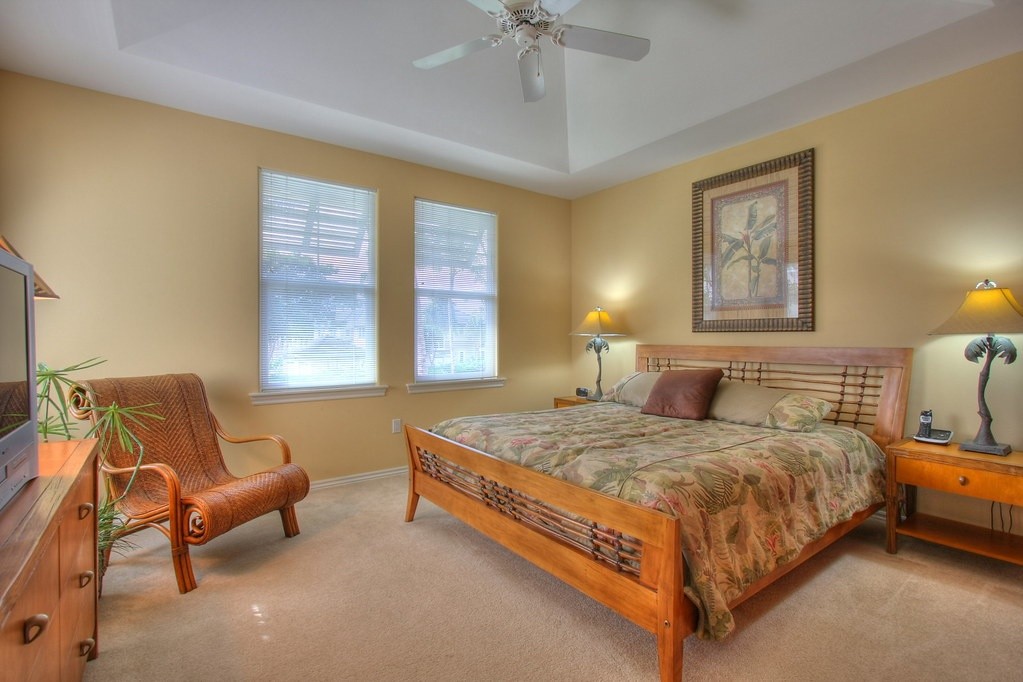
[[69, 372, 310, 595]]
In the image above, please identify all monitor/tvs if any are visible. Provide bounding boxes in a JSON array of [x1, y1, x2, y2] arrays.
[[0, 249, 41, 511]]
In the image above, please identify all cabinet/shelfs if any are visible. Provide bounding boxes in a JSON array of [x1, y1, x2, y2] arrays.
[[0, 436, 104, 682]]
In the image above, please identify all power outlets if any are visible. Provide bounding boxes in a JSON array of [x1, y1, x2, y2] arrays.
[[393, 418, 402, 434]]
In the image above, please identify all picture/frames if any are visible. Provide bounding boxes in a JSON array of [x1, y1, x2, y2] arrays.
[[691, 145, 816, 332]]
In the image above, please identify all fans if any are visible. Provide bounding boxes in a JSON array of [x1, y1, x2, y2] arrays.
[[412, 0, 650, 102]]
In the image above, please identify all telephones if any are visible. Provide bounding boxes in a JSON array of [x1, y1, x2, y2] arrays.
[[913, 409, 953, 445]]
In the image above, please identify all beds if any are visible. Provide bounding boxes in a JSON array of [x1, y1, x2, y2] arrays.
[[402, 341, 923, 681]]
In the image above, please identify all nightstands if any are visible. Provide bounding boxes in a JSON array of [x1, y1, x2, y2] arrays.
[[883, 438, 1023, 578], [554, 395, 597, 409]]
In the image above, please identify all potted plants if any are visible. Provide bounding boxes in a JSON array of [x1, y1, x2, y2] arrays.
[[29, 358, 147, 597]]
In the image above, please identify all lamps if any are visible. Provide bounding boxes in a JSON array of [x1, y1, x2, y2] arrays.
[[927, 280, 1023, 455], [569, 308, 625, 401]]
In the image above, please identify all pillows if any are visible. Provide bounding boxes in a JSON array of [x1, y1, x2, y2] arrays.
[[641, 366, 722, 421], [600, 373, 834, 434]]
